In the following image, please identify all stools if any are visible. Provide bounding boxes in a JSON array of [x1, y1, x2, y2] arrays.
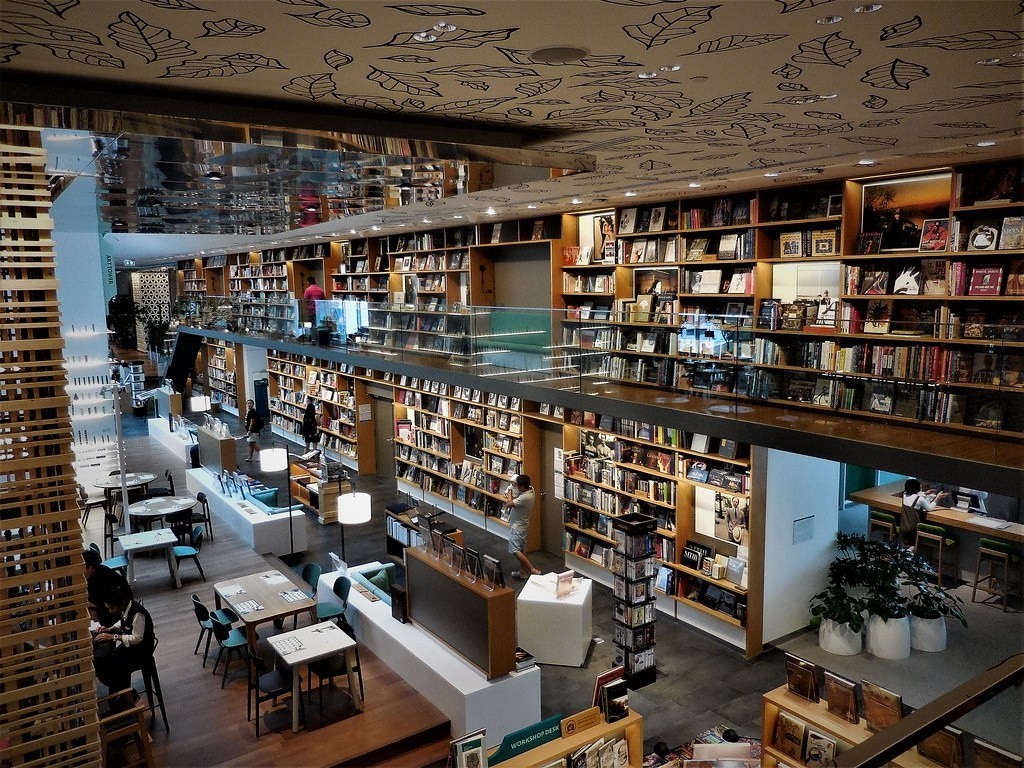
[[971, 538, 1024, 613], [914, 522, 959, 589], [868, 511, 900, 542]]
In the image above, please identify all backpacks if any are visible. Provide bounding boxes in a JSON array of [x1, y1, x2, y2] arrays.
[[304, 412, 317, 436], [252, 409, 265, 438]]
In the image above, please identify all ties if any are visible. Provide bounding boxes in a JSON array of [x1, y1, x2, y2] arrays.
[[735, 508, 737, 519]]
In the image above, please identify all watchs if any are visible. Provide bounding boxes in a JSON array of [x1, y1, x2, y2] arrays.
[[113, 634, 118, 642]]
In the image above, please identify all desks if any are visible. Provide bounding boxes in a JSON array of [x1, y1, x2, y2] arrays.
[[129, 495, 198, 559], [118, 528, 182, 589], [92, 472, 158, 514], [214, 569, 317, 684], [266, 621, 361, 733]]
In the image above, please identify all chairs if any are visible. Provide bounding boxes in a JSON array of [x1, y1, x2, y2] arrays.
[[208, 611, 260, 689], [280, 563, 321, 630], [306, 620, 364, 708], [149, 468, 176, 497], [108, 633, 169, 732], [309, 577, 350, 624], [128, 494, 164, 531], [173, 526, 206, 583], [117, 486, 145, 527], [190, 593, 239, 669], [246, 644, 308, 738], [77, 484, 109, 529], [165, 508, 194, 546], [181, 492, 214, 541], [98, 689, 154, 768], [89, 543, 129, 579], [104, 514, 141, 561]]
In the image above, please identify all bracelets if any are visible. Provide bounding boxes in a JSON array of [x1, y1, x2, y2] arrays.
[[248, 430, 251, 431]]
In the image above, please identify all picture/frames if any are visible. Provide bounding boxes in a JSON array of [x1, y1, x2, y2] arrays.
[[592, 213, 615, 260]]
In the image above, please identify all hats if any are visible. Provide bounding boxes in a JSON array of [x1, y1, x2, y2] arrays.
[[732, 526, 742, 542], [510, 474, 519, 482]]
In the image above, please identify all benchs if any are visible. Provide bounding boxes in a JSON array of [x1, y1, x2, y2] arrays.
[[351, 562, 396, 606], [474, 311, 551, 374]]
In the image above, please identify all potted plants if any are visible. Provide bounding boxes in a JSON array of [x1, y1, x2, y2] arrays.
[[901, 580, 969, 653], [860, 585, 911, 659], [143, 320, 158, 360], [152, 322, 171, 363], [807, 586, 867, 655], [827, 530, 937, 635]]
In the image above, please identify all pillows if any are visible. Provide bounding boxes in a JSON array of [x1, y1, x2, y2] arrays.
[[191, 430, 197, 436], [255, 491, 278, 507]]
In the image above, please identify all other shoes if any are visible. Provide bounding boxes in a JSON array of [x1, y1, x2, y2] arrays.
[[246, 459, 252, 462], [256, 460, 260, 462], [536, 571, 541, 575], [511, 571, 529, 580]]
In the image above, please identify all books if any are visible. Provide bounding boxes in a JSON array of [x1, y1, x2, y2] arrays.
[[103, 147, 467, 237], [171, 185, 1024, 768]]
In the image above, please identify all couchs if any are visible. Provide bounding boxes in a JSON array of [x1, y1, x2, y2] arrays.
[[369, 568, 390, 595], [245, 487, 303, 515]]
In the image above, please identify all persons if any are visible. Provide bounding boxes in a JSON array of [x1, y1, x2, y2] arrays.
[[508, 475, 541, 580], [304, 277, 325, 320], [302, 403, 321, 454], [245, 399, 263, 462], [900, 479, 949, 545], [504, 474, 520, 509], [0, 542, 156, 743]]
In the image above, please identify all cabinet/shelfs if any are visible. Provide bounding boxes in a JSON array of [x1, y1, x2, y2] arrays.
[[289, 460, 352, 527], [486, 707, 644, 768], [760, 683, 944, 768], [556, 166, 1024, 442], [384, 509, 464, 570], [200, 337, 251, 421], [265, 349, 394, 478], [561, 424, 768, 660], [392, 374, 541, 554], [174, 219, 563, 379]]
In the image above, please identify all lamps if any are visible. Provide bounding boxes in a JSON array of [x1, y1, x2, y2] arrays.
[[259, 440, 301, 567], [190, 393, 211, 425], [337, 473, 372, 563]]
